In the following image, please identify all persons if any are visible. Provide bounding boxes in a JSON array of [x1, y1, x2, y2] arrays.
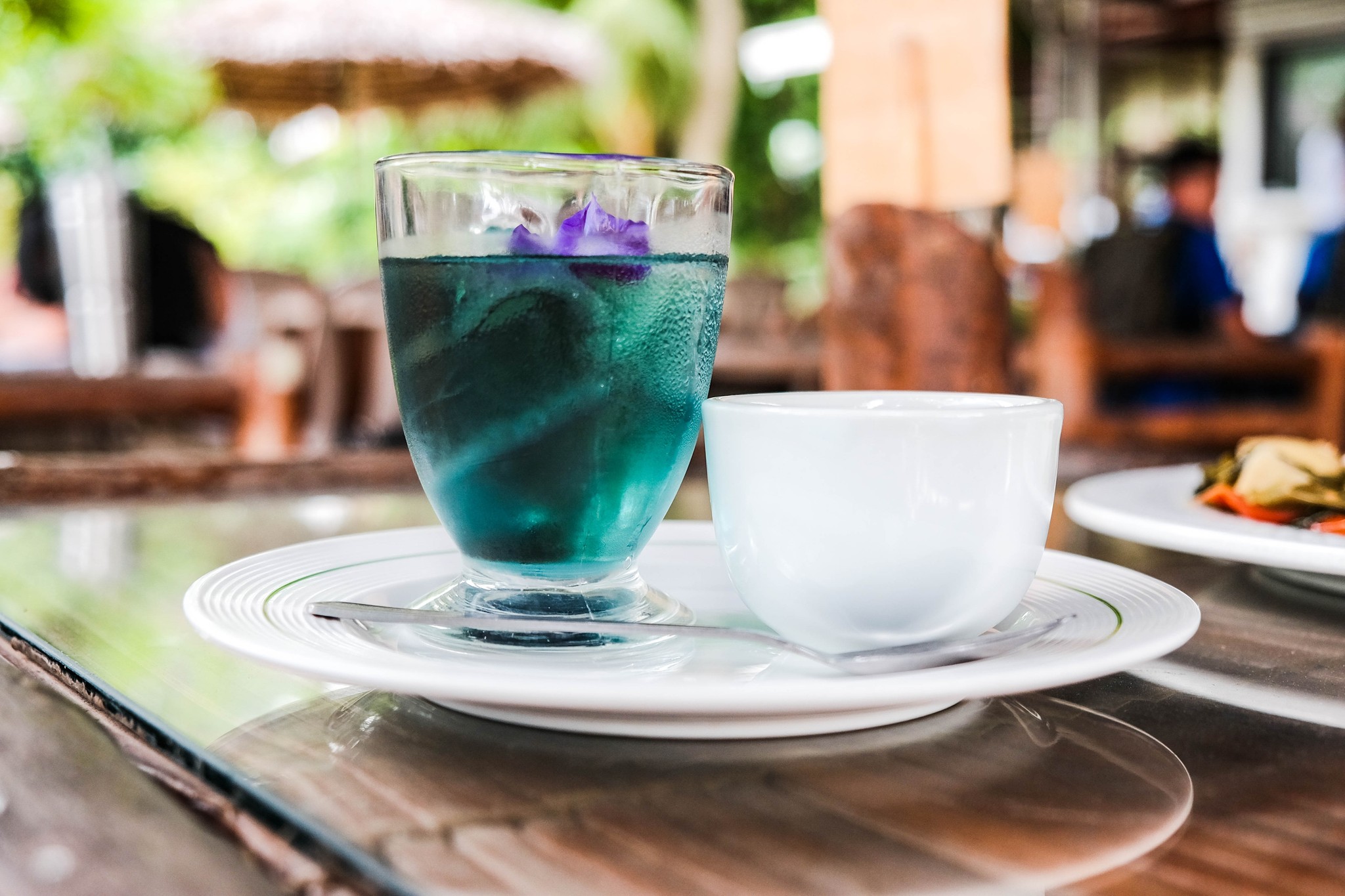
[[1086, 145, 1168, 419], [1147, 138, 1267, 362], [1287, 94, 1344, 329]]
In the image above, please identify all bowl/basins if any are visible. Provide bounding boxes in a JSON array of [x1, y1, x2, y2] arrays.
[[701, 388, 1064, 649]]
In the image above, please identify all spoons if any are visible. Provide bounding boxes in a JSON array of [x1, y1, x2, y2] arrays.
[[305, 598, 1080, 674]]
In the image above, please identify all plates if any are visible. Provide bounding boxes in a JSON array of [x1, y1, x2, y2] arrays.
[[206, 688, 1194, 895], [180, 525, 1203, 744], [1064, 467, 1345, 588], [1116, 564, 1344, 729]]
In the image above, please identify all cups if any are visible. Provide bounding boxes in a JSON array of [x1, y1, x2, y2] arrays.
[[370, 145, 736, 584]]
